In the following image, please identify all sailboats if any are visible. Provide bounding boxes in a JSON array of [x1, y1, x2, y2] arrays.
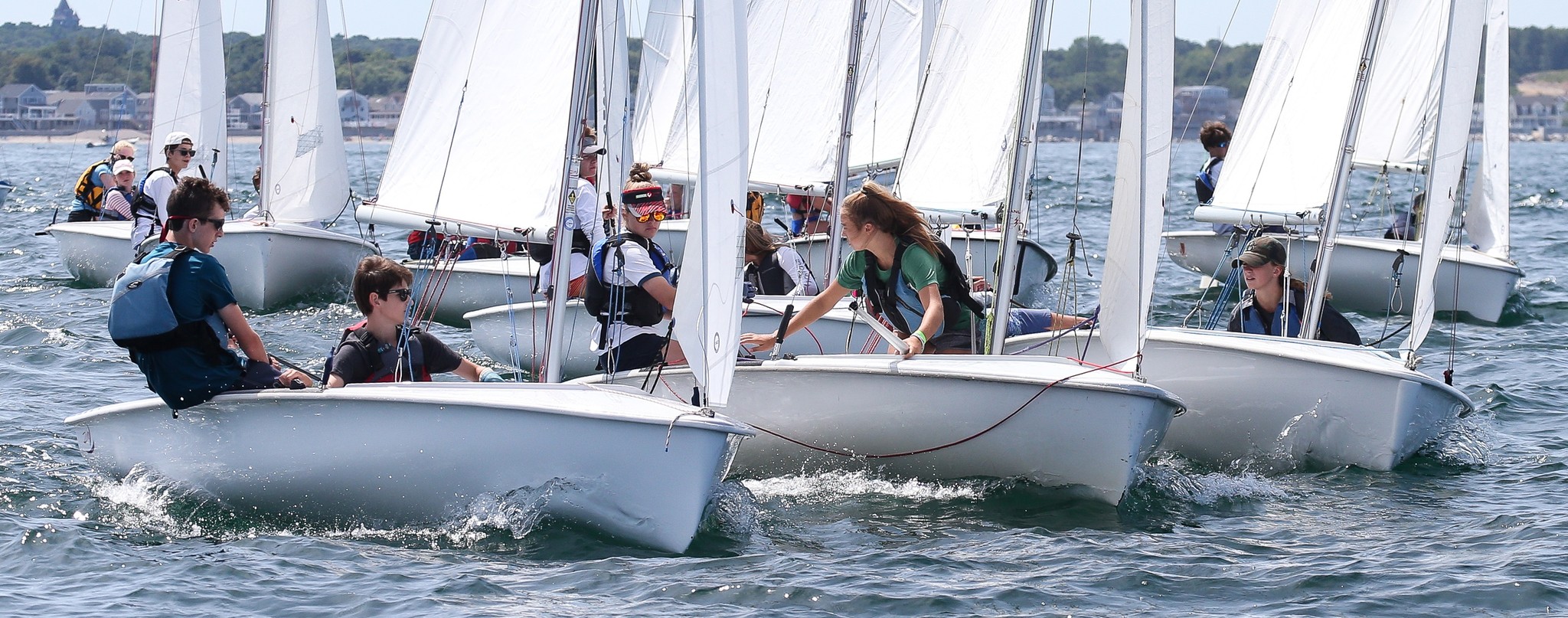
[[1005, 0, 1474, 472], [65, 0, 755, 553], [135, 1, 381, 311], [352, 0, 1190, 506], [1160, 0, 1527, 327], [35, 0, 237, 288]]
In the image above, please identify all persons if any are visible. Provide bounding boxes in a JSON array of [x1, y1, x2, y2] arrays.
[[590, 162, 689, 375], [67, 139, 134, 223], [538, 126, 606, 300], [1384, 192, 1426, 241], [787, 193, 832, 235], [278, 255, 506, 388], [101, 158, 136, 221], [964, 274, 1094, 339], [746, 219, 820, 296], [740, 181, 985, 354], [1198, 121, 1299, 234], [1227, 235, 1362, 347], [130, 132, 194, 252], [665, 183, 683, 214], [107, 176, 282, 410]]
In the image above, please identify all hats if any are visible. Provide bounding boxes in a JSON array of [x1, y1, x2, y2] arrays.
[[158, 132, 193, 154], [1230, 236, 1287, 268], [113, 160, 134, 176], [582, 137, 607, 156], [622, 187, 668, 217]]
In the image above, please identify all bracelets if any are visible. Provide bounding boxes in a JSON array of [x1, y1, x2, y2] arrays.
[[913, 330, 926, 344], [910, 334, 925, 354], [478, 367, 493, 381]]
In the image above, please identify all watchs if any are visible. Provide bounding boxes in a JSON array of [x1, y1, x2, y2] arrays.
[[265, 351, 274, 366]]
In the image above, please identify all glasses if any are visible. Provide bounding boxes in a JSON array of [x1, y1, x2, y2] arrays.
[[1219, 140, 1231, 147], [377, 288, 411, 302], [636, 212, 666, 223], [191, 215, 225, 231], [118, 154, 135, 162], [170, 149, 196, 157]]
